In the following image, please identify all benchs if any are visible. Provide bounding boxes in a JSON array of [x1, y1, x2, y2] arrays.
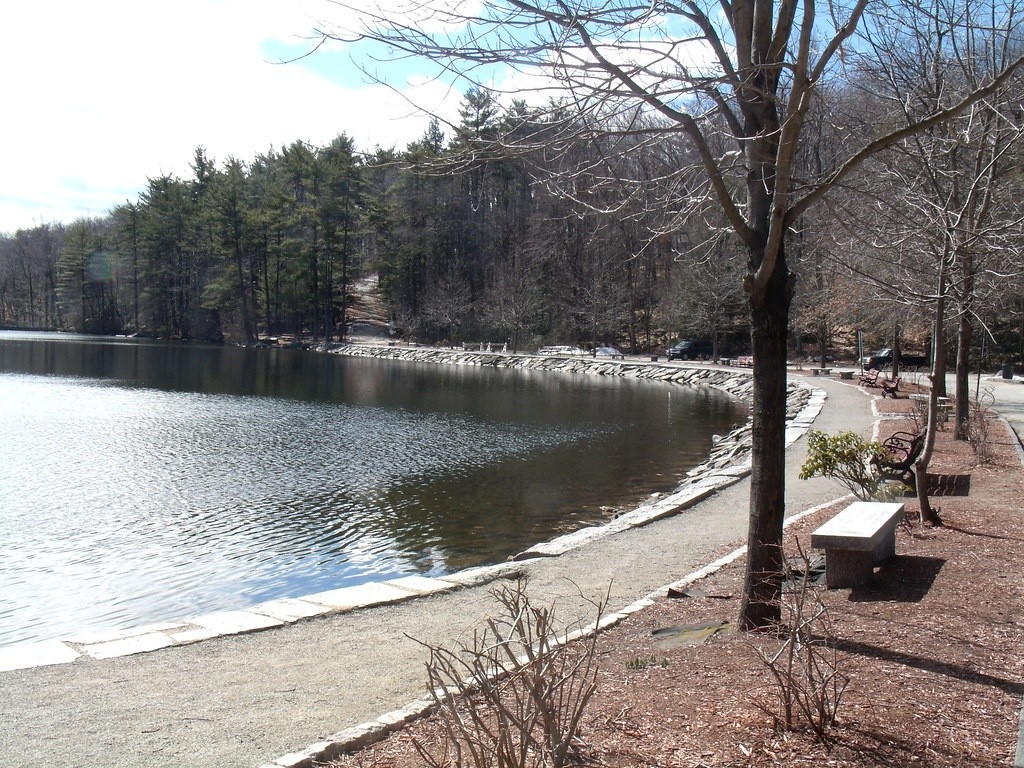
[[810, 368, 832, 375], [868, 426, 927, 495], [610, 354, 628, 360], [910, 393, 954, 421], [731, 356, 750, 368], [810, 501, 906, 589], [839, 371, 855, 379], [858, 368, 881, 387], [881, 376, 901, 398]]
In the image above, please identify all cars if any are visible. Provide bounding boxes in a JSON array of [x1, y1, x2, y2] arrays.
[[538, 346, 622, 356]]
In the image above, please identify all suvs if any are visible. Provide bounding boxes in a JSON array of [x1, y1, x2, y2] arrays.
[[665, 339, 713, 361]]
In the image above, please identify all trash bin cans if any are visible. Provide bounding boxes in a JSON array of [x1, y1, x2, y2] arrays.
[[1002, 361, 1015, 379]]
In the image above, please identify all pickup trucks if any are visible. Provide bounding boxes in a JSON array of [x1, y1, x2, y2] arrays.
[[857, 348, 927, 372]]
